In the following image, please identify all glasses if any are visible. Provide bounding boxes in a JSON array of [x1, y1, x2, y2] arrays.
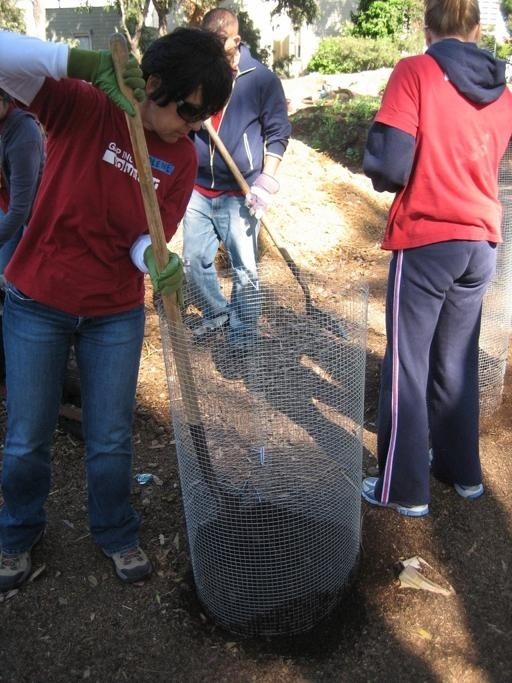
[[174, 96, 211, 124]]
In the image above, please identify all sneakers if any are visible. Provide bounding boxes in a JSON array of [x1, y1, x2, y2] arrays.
[[193, 312, 230, 337], [362, 475, 430, 519], [430, 448, 485, 500], [102, 542, 154, 586], [0, 545, 48, 600]]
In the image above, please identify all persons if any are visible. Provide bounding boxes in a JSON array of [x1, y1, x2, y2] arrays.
[[0, 86, 46, 404], [0, 21, 237, 598], [358, 0, 512, 516], [174, 5, 291, 370]]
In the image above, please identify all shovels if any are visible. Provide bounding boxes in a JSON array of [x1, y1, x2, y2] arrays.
[[202, 119, 347, 338], [110, 32, 256, 579]]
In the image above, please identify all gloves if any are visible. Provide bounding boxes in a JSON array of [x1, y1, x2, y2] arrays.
[[243, 173, 282, 219], [66, 46, 144, 117], [144, 244, 186, 305]]
